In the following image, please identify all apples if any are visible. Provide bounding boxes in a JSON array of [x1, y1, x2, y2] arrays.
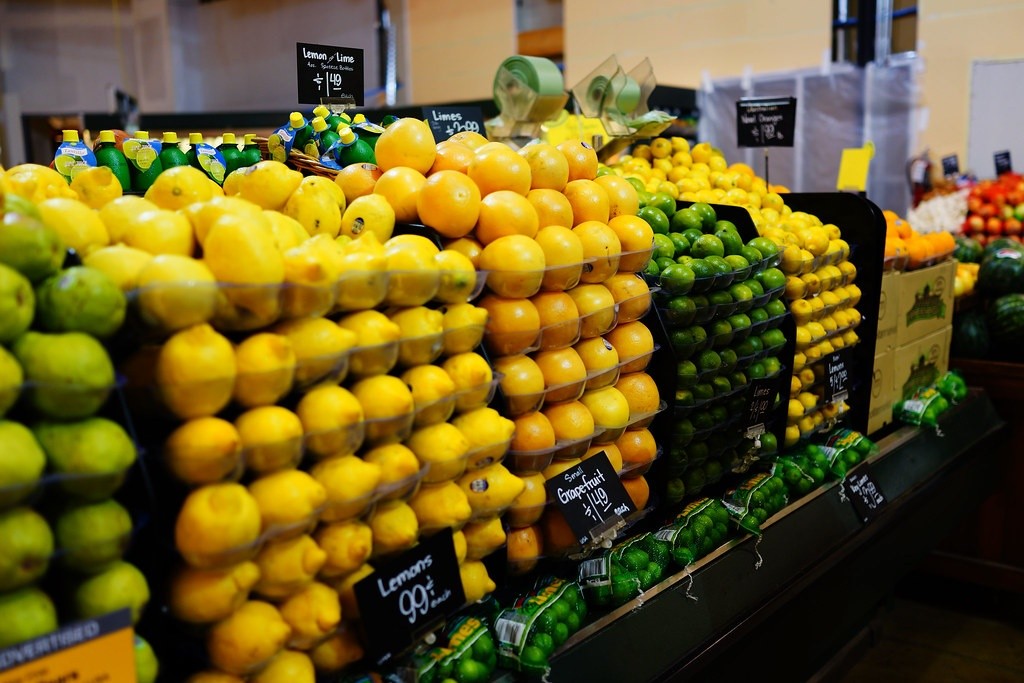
[[955, 172, 1024, 245]]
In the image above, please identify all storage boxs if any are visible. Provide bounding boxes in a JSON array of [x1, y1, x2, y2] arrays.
[[866, 258, 958, 443]]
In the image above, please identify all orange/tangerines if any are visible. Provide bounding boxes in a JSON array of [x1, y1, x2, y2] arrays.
[[882, 211, 956, 260], [334, 118, 659, 574]]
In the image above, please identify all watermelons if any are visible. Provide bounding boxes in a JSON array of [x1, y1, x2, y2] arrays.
[[952, 237, 1024, 362]]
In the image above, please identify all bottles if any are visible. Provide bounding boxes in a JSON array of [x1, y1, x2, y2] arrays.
[[53, 103, 402, 197]]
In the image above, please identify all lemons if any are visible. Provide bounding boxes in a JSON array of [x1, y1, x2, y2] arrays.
[[412, 428, 873, 683], [896, 371, 967, 425], [0, 159, 527, 683], [597, 166, 786, 506], [612, 136, 862, 445]]
